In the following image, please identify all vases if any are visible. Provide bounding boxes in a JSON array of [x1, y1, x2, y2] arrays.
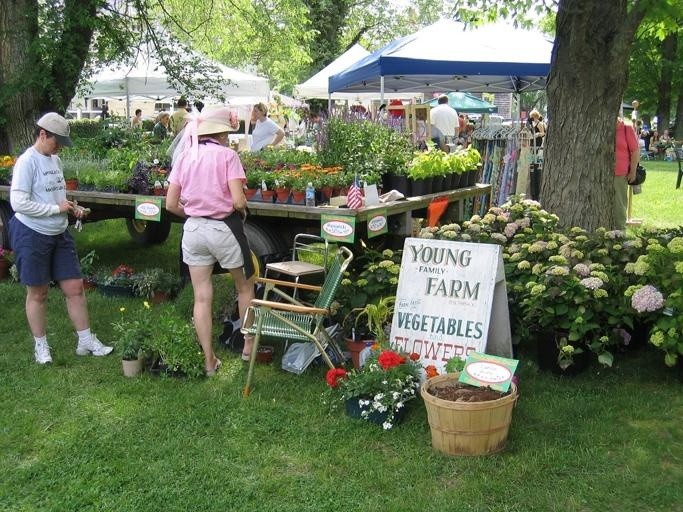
[[343, 393, 406, 425]]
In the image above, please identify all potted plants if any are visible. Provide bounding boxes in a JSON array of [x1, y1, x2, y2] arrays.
[[0, 245, 206, 378]]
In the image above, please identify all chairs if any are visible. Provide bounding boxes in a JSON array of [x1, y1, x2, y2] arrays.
[[240, 246, 356, 397], [264, 233, 328, 304], [638, 140, 656, 161], [675, 152, 683, 189]]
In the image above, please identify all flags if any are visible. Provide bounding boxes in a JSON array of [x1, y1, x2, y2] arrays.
[[346, 174, 363, 208]]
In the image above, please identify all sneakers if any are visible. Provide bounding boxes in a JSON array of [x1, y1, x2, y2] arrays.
[[74, 338, 116, 357], [33, 342, 56, 365], [241, 344, 276, 363], [203, 357, 225, 377]]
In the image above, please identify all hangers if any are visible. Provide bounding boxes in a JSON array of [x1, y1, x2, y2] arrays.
[[471, 121, 533, 141]]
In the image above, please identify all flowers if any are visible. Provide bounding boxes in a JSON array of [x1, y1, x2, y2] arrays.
[[326, 297, 439, 429]]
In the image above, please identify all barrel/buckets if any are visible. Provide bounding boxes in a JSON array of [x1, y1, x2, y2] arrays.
[[358, 342, 374, 368], [420, 372, 518, 458]]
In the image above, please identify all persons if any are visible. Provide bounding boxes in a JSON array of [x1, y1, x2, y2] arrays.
[[8, 112, 114, 365], [637, 121, 671, 159], [165, 104, 275, 376], [613, 117, 640, 227], [133, 96, 546, 156]]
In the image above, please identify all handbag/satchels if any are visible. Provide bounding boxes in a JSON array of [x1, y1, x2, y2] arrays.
[[624, 163, 648, 186]]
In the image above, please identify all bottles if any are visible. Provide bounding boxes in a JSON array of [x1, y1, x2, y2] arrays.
[[305, 182, 315, 208]]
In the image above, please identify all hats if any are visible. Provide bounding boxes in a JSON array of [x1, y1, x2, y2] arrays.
[[193, 103, 242, 137], [34, 111, 75, 148]]
